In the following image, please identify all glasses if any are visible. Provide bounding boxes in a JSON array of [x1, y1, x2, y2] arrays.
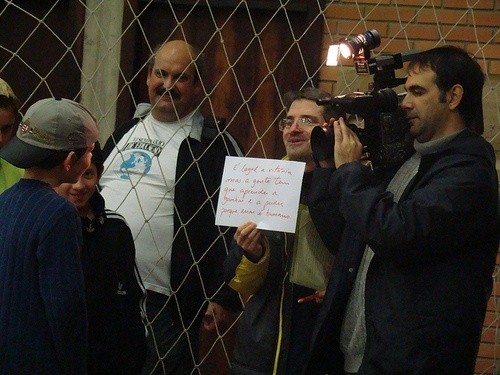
[[278, 117, 325, 132]]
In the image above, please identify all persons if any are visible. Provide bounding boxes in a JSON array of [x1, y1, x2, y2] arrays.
[[99, 40, 247, 375], [0, 78, 26, 197], [318, 46, 500, 375], [54, 139, 148, 375], [0, 98, 98, 375], [233, 87, 353, 375]]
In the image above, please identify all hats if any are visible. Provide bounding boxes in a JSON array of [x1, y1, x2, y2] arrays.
[[0, 77, 15, 99], [0, 97, 100, 169]]
